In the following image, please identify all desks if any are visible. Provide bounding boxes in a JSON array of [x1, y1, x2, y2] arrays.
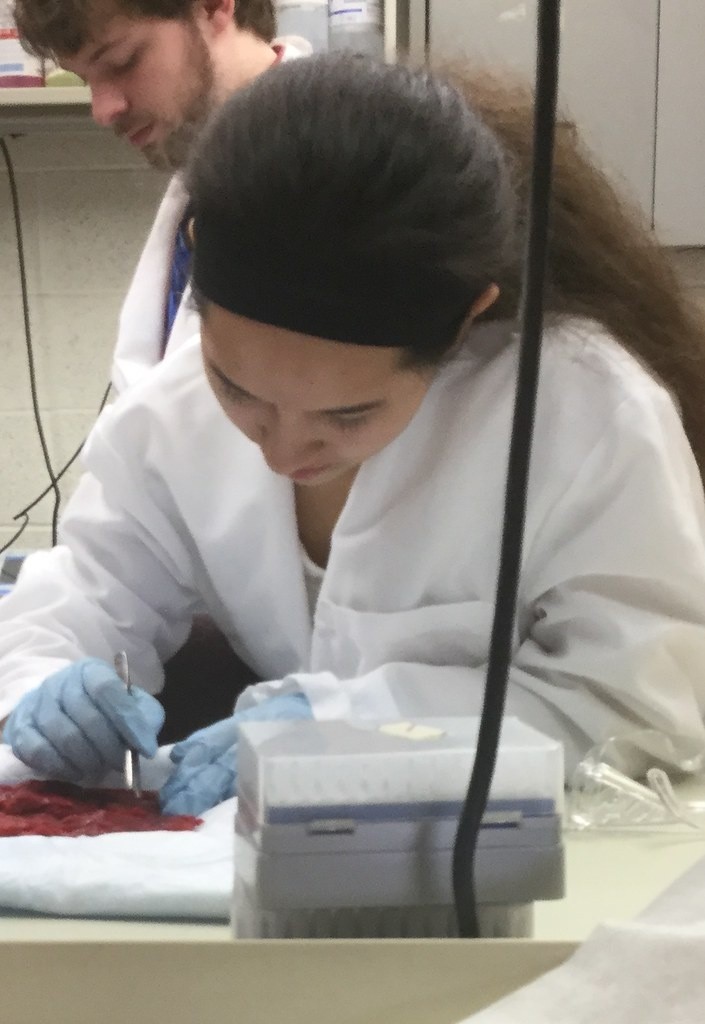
[[3, 775, 703, 1024]]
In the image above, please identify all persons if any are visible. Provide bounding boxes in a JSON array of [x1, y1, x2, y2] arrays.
[[9, 2, 315, 393], [2, 51, 705, 817]]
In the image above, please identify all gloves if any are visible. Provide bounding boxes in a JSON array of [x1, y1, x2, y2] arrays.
[[1, 654, 154, 785], [151, 688, 313, 821]]
